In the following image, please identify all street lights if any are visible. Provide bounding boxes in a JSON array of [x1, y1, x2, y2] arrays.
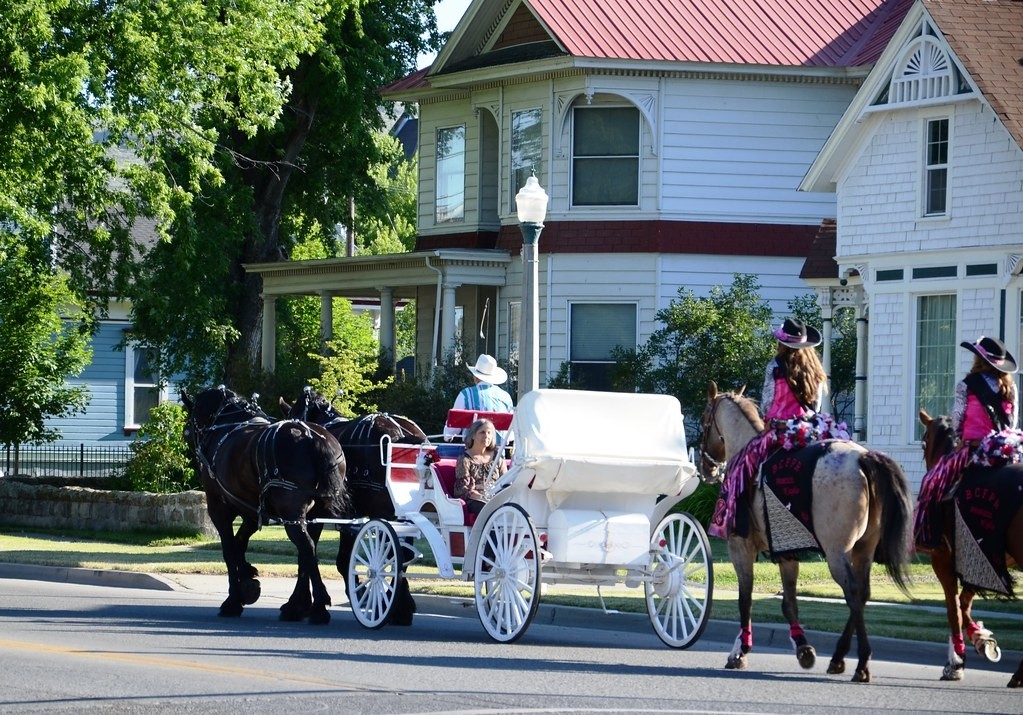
[[514, 163, 552, 403]]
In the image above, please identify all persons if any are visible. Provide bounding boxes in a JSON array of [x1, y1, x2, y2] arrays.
[[454, 418, 508, 516], [913, 335, 1023, 551], [708, 318, 828, 541], [443, 353, 513, 459]]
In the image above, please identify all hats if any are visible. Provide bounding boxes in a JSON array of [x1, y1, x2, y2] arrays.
[[770, 318, 822, 349], [466, 354, 508, 384], [960, 335, 1019, 373]]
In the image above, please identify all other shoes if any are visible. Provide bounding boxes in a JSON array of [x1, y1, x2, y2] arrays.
[[483, 558, 494, 571]]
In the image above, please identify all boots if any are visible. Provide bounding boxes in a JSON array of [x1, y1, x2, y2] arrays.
[[917, 501, 946, 553], [730, 489, 750, 540]]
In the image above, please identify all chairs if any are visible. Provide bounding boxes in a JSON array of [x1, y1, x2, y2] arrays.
[[446, 409, 515, 448]]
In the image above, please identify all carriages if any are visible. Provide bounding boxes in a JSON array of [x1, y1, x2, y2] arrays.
[[176, 380, 715, 650]]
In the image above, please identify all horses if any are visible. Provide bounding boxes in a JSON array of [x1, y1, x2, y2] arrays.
[[696, 379, 913, 683], [913, 409, 1023, 689], [274, 388, 439, 627], [179, 381, 348, 627]]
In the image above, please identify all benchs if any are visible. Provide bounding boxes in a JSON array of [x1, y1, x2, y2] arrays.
[[429, 459, 514, 526]]
